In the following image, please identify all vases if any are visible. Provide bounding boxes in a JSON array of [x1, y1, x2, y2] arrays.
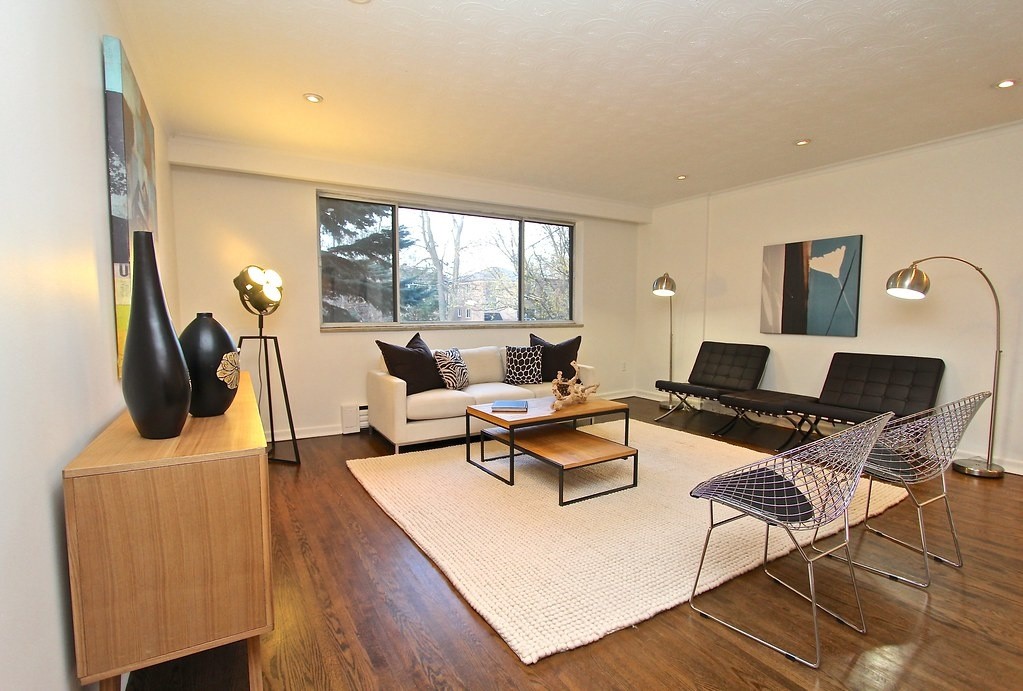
[[121, 230, 192, 440], [178, 312, 240, 418]]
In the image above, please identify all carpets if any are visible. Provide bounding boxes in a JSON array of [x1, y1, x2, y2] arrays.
[[346, 418, 909, 665]]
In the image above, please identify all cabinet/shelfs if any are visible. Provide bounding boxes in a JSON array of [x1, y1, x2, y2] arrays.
[[61, 370, 274, 691]]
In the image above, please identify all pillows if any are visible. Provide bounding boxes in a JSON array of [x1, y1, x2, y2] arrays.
[[434, 346, 469, 391], [504, 345, 544, 386], [529, 333, 583, 386], [375, 332, 444, 396]]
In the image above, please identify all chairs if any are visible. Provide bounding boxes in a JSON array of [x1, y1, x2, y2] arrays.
[[811, 391, 993, 589], [688, 410, 895, 668]]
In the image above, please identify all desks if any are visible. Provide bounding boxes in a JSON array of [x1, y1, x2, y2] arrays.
[[480, 423, 638, 506]]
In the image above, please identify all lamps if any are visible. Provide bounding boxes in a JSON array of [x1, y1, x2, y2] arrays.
[[886, 256, 1005, 478], [653, 273, 683, 411]]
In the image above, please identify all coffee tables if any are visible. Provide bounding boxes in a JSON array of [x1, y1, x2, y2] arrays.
[[465, 396, 630, 487]]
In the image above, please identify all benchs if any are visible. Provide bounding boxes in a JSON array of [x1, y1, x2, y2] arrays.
[[718, 389, 818, 453], [654, 341, 770, 436], [774, 353, 945, 483]]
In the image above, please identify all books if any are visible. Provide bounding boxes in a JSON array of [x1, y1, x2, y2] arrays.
[[491, 400, 528, 412]]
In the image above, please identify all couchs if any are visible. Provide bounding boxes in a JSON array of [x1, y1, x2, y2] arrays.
[[367, 345, 596, 456]]
